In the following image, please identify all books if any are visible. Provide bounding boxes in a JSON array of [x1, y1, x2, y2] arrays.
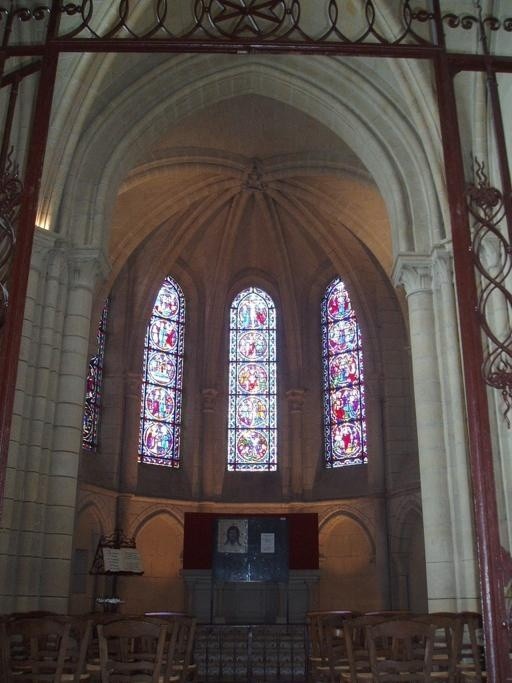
[[101, 545, 145, 573]]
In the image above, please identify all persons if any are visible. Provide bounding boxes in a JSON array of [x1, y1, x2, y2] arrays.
[[223, 523, 244, 548]]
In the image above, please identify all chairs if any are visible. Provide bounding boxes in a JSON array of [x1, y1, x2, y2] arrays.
[[305, 611, 486, 683], [1, 611, 198, 683]]
